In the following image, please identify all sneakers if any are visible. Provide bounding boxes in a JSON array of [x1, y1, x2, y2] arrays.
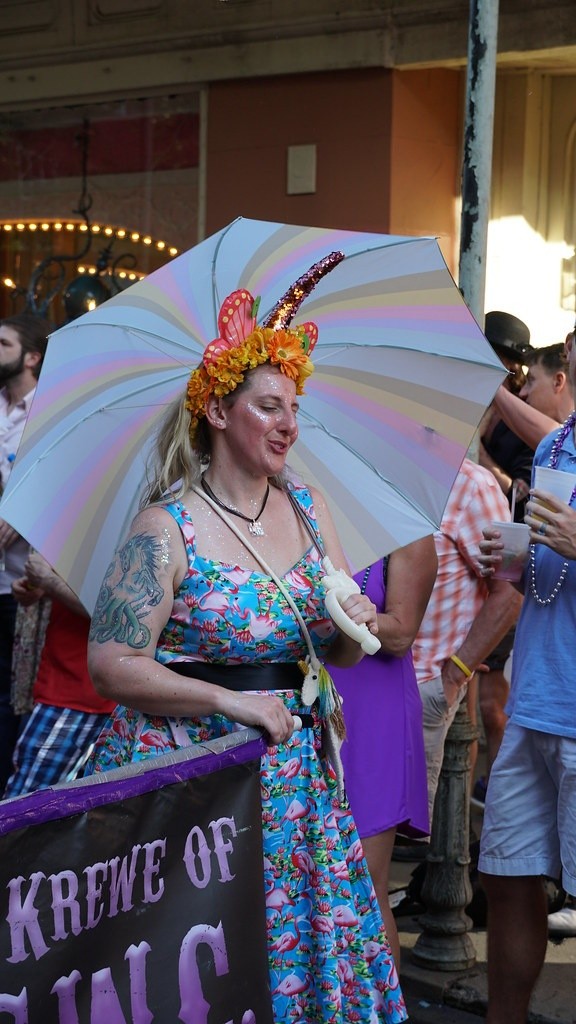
[[472, 777, 486, 811], [547, 907, 576, 936]]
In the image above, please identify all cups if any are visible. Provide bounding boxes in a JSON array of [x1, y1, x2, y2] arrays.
[[530, 465, 576, 525], [490, 522, 531, 582]]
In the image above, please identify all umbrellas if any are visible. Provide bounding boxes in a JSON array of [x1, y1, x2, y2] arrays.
[[1, 216, 514, 656]]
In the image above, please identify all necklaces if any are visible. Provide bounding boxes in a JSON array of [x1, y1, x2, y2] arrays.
[[202, 470, 270, 536], [361, 566, 370, 595], [529, 412, 575, 605]]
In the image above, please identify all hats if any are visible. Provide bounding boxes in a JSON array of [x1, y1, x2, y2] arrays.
[[487, 310, 538, 365]]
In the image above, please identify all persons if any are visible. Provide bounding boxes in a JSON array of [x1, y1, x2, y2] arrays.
[[0, 312, 575, 1023]]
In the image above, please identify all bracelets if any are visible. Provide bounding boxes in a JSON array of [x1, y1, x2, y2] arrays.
[[451, 655, 472, 677]]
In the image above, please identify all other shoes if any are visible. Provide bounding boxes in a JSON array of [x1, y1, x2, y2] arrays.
[[391, 862, 426, 916], [392, 836, 429, 864]]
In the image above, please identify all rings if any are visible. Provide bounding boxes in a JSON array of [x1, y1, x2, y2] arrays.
[[539, 523, 547, 533]]
